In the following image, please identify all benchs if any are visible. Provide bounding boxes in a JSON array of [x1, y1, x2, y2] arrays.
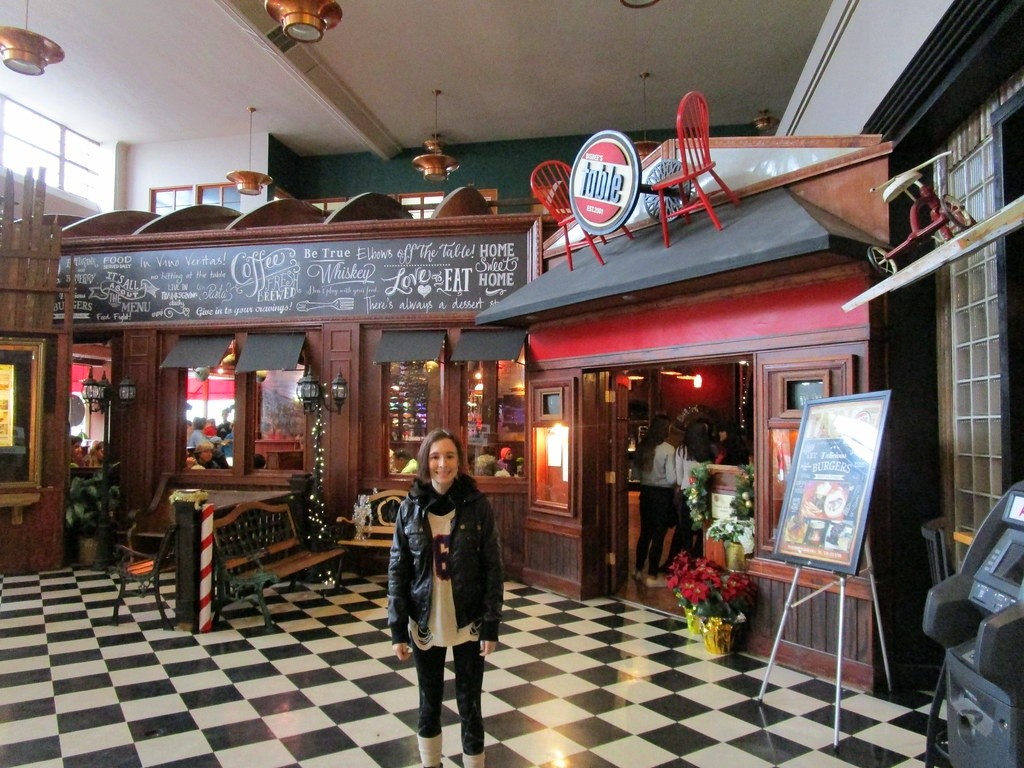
[[113, 528, 177, 632], [126, 472, 291, 561], [212, 501, 347, 632], [337, 489, 411, 595]]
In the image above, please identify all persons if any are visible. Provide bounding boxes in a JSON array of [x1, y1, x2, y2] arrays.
[[468, 445, 500, 476], [675, 422, 713, 558], [498, 448, 517, 473], [71, 435, 104, 466], [632, 415, 677, 588], [714, 420, 749, 466], [387, 428, 504, 768], [187, 409, 234, 468], [393, 449, 418, 473]]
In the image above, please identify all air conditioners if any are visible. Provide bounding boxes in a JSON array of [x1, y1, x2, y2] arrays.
[[633, 72, 662, 164]]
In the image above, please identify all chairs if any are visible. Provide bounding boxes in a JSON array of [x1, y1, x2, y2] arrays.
[[652, 93, 739, 246], [529, 159, 634, 268]]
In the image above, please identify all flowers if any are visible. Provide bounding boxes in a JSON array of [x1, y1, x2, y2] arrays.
[[666, 460, 756, 619]]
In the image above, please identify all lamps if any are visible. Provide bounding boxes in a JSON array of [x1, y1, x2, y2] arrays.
[[227, 107, 272, 195], [0, 0, 65, 75], [264, 0, 343, 43], [413, 88, 461, 183], [297, 366, 347, 584], [83, 367, 136, 570], [754, 108, 777, 129]]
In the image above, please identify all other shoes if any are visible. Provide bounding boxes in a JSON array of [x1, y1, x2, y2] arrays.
[[642, 575, 670, 588], [634, 570, 645, 580]]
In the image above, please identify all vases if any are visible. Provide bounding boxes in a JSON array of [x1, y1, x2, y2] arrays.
[[698, 616, 738, 654], [725, 541, 746, 572], [682, 603, 703, 635]]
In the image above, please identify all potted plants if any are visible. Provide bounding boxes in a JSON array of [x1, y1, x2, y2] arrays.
[[65, 472, 120, 564]]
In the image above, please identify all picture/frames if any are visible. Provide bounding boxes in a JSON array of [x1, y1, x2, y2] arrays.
[[772, 389, 892, 574], [0, 337, 47, 489]]
